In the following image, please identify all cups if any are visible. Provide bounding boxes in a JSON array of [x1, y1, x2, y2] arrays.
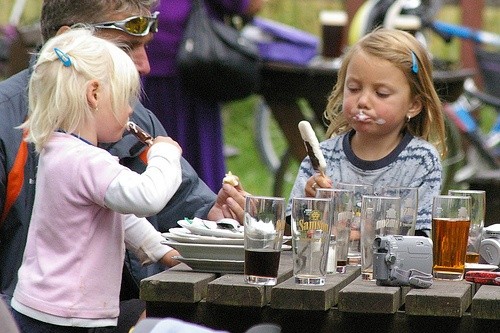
[[320, 11, 347, 60], [447, 190, 486, 264], [243, 196, 285, 286], [431, 196, 472, 281], [315, 188, 354, 274], [291, 198, 334, 285], [335, 182, 373, 266], [382, 187, 418, 236], [361, 196, 400, 281]]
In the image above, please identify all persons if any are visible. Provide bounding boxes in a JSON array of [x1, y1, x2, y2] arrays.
[[286, 28, 444, 253], [0, 0, 260, 330], [12, 29, 183, 333], [137, 0, 264, 196]]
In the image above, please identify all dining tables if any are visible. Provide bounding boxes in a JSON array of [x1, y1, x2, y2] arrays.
[[140, 246, 500, 333], [244, 58, 474, 216]]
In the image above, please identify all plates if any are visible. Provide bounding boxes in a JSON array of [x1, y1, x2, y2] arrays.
[[463, 263, 499, 279], [161, 219, 292, 273]]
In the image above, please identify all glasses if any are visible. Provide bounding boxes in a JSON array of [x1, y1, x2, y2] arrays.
[[71, 10, 160, 36]]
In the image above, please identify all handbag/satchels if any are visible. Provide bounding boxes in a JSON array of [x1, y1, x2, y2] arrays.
[[176, 0, 265, 102]]
